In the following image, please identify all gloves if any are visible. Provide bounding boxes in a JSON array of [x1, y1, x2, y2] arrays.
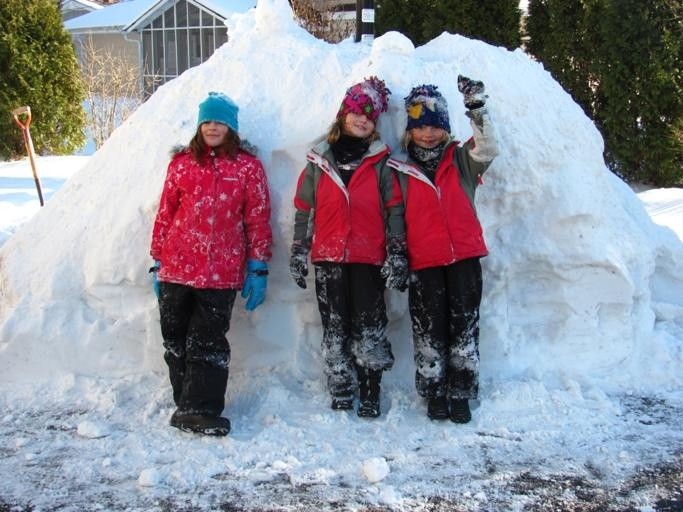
[[153, 260, 160, 299], [381, 234, 411, 292], [241, 260, 269, 313], [457, 73, 487, 107], [290, 242, 311, 289]]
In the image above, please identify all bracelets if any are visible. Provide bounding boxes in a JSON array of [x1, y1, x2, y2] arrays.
[[149, 267, 160, 273], [250, 270, 269, 276]]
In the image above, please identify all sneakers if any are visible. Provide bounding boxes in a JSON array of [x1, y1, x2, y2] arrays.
[[331, 390, 354, 409], [356, 400, 382, 418], [427, 397, 450, 420], [168, 398, 231, 438], [450, 398, 472, 424]]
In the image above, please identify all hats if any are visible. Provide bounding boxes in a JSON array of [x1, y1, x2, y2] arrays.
[[197, 89, 240, 133], [337, 74, 392, 125], [403, 82, 451, 134]]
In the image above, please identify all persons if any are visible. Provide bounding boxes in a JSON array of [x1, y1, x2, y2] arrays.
[[383, 74, 501, 425], [147, 89, 273, 437], [289, 76, 411, 417]]
